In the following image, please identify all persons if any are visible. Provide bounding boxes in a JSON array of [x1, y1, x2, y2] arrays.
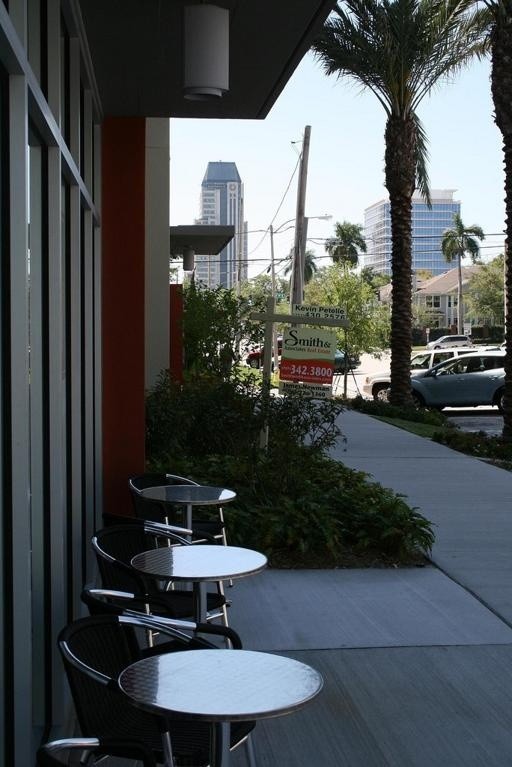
[[219, 344, 237, 382]]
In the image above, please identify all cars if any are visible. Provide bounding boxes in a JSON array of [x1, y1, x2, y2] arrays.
[[247, 335, 361, 374], [363, 333, 506, 414]]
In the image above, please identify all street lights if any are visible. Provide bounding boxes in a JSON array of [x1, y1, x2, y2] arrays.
[[268, 213, 334, 375]]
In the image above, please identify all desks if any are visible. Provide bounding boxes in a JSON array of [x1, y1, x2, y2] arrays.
[[130, 543, 269, 639], [116, 647, 325, 766], [117, 543, 324, 767], [137, 483, 237, 543]]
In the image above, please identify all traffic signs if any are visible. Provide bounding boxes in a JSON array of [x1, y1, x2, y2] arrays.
[[274, 293, 285, 299]]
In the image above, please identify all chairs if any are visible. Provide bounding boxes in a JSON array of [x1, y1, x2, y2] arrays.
[[56, 614, 256, 766], [82, 581, 244, 651], [126, 473, 238, 589], [101, 510, 219, 545], [90, 524, 238, 649], [32, 733, 164, 767]]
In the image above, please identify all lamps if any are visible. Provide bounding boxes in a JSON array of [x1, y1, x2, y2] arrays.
[[180, 1, 231, 103]]
